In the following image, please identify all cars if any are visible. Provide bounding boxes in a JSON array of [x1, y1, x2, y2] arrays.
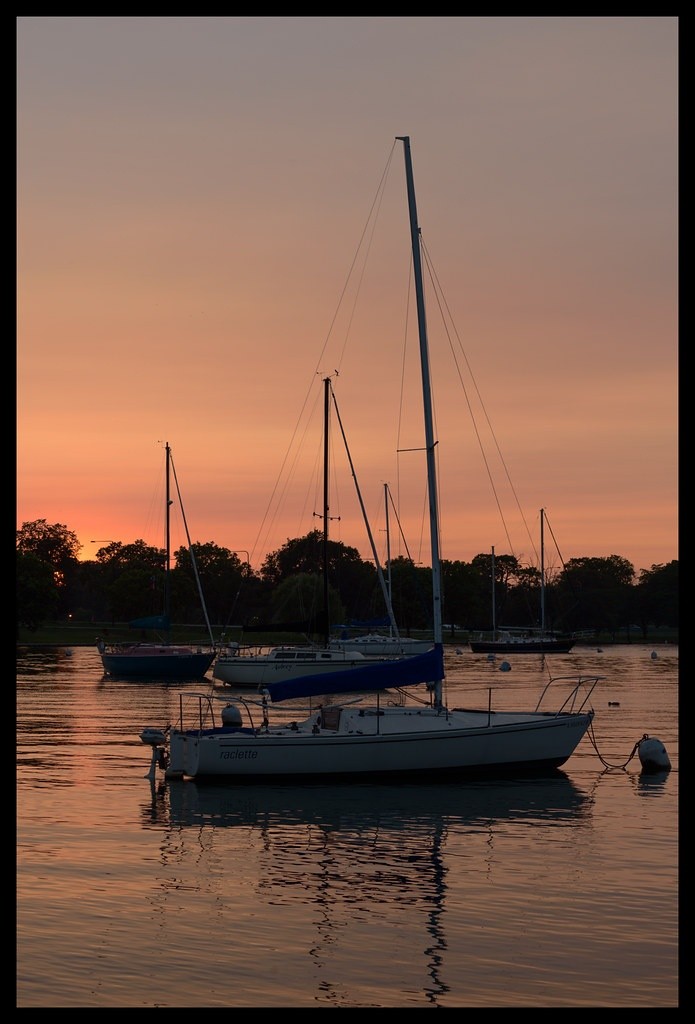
[[630, 624, 641, 631], [442, 624, 461, 630]]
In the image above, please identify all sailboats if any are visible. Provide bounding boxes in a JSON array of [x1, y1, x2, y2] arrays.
[[328, 482, 435, 655], [211, 369, 405, 690], [139, 135, 608, 789], [93, 438, 219, 684], [468, 507, 583, 653]]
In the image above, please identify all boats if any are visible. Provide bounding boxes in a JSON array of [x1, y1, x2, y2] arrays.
[[572, 630, 604, 644]]
